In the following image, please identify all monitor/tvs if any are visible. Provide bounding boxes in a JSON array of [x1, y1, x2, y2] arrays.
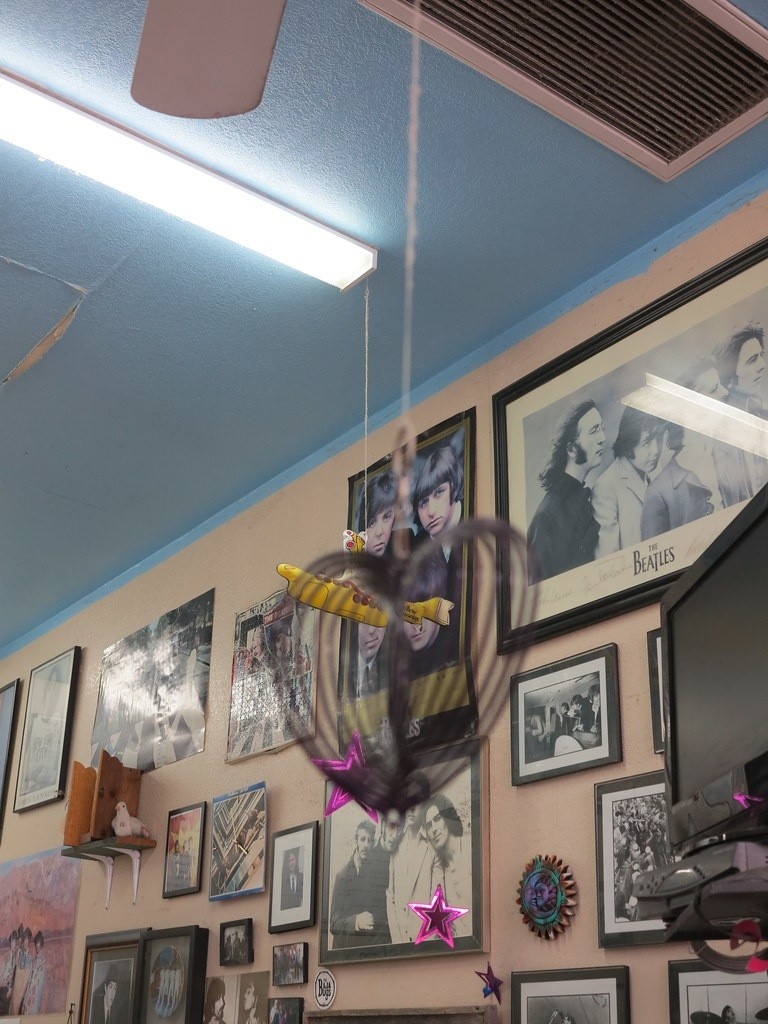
[[660, 481, 768, 854]]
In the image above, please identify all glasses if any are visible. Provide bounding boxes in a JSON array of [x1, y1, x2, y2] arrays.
[[575, 423, 606, 439]]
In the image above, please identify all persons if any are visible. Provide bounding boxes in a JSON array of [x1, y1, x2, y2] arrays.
[[167, 836, 198, 892], [528, 320, 768, 587], [150, 959, 183, 1010], [349, 445, 466, 698], [239, 809, 265, 849], [90, 974, 125, 1024], [721, 1006, 741, 1024], [525, 882, 550, 913], [524, 684, 601, 764], [19, 665, 64, 797], [612, 793, 674, 923], [224, 931, 247, 961], [0, 924, 46, 1016], [560, 1012, 575, 1024], [238, 621, 312, 698], [330, 772, 472, 950], [269, 999, 290, 1024], [274, 948, 300, 981], [281, 850, 303, 910], [244, 979, 263, 1024], [203, 978, 226, 1024]]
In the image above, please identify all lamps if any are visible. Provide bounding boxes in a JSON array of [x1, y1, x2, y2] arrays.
[[0, 68, 380, 296], [618, 372, 768, 467]]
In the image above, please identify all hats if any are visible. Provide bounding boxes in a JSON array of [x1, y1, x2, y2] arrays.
[[105, 965, 120, 984]]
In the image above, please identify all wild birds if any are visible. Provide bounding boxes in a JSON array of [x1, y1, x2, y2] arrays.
[[110, 801, 148, 838]]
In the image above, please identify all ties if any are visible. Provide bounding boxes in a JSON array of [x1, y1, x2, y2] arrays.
[[292, 876, 294, 894], [362, 666, 369, 697]]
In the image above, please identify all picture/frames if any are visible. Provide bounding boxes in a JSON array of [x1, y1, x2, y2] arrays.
[[132, 922, 211, 1024], [510, 964, 632, 1024], [208, 778, 270, 902], [319, 732, 494, 973], [0, 675, 24, 845], [272, 941, 308, 987], [589, 766, 675, 951], [646, 627, 668, 757], [12, 642, 85, 815], [160, 799, 207, 899], [266, 995, 305, 1024], [489, 234, 768, 662], [331, 402, 483, 765], [76, 926, 153, 1024], [218, 916, 254, 967], [267, 817, 321, 935], [508, 640, 624, 787], [667, 953, 768, 1024]]
